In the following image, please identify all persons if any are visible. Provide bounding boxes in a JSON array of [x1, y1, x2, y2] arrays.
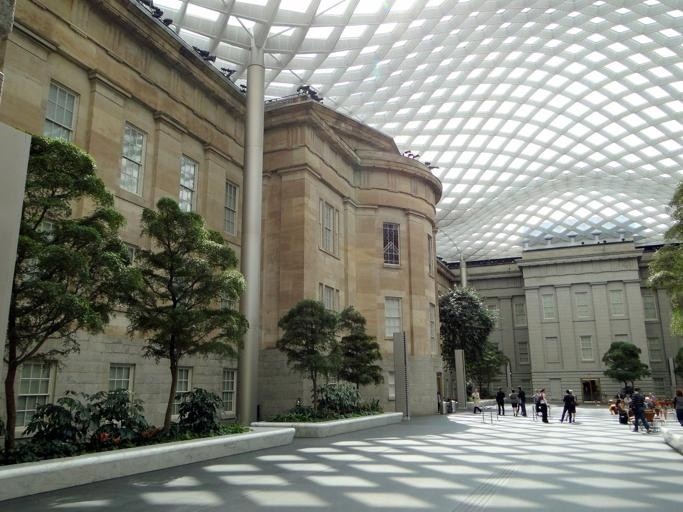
[[437, 389, 441, 413], [469, 385, 682, 434]]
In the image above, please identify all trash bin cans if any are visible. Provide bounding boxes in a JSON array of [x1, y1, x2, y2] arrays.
[[440, 401, 446, 414], [450, 400, 456, 413]]
